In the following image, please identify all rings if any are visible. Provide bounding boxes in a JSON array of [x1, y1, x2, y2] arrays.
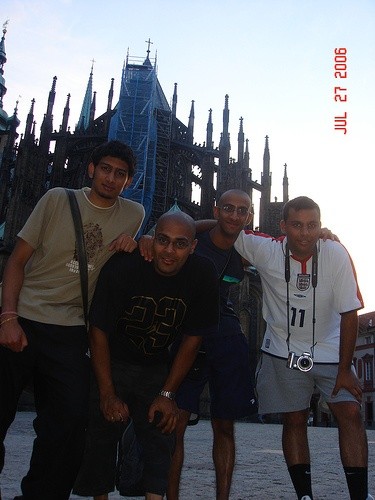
[[111, 416, 114, 420]]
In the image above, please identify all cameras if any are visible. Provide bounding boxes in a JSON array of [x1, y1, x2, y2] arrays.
[[286, 351, 313, 373]]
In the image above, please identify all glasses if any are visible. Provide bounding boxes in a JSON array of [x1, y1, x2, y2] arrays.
[[217, 204, 254, 218]]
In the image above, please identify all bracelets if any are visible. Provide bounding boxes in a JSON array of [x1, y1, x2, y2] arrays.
[[0, 311, 20, 325]]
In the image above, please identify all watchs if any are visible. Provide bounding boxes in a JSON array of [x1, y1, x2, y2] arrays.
[[159, 389, 176, 402]]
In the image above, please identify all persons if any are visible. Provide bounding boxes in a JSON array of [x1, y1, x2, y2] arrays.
[[86, 210, 221, 500], [138, 188, 341, 500], [0, 140, 146, 500], [234, 194, 369, 500]]
